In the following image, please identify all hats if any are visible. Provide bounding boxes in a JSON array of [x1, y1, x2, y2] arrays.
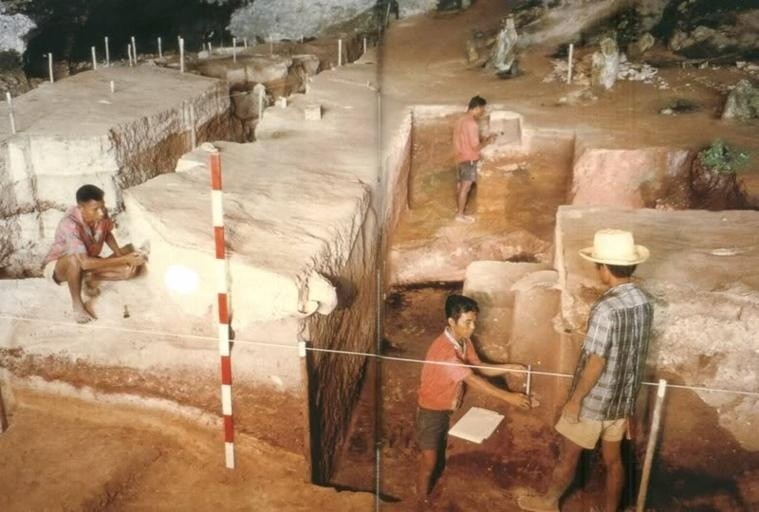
[[578, 227, 650, 267]]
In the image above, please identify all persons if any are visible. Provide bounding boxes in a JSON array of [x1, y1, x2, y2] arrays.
[[517, 227, 653, 512], [43, 184, 145, 323], [453, 95, 496, 223], [415, 295, 531, 502]]
